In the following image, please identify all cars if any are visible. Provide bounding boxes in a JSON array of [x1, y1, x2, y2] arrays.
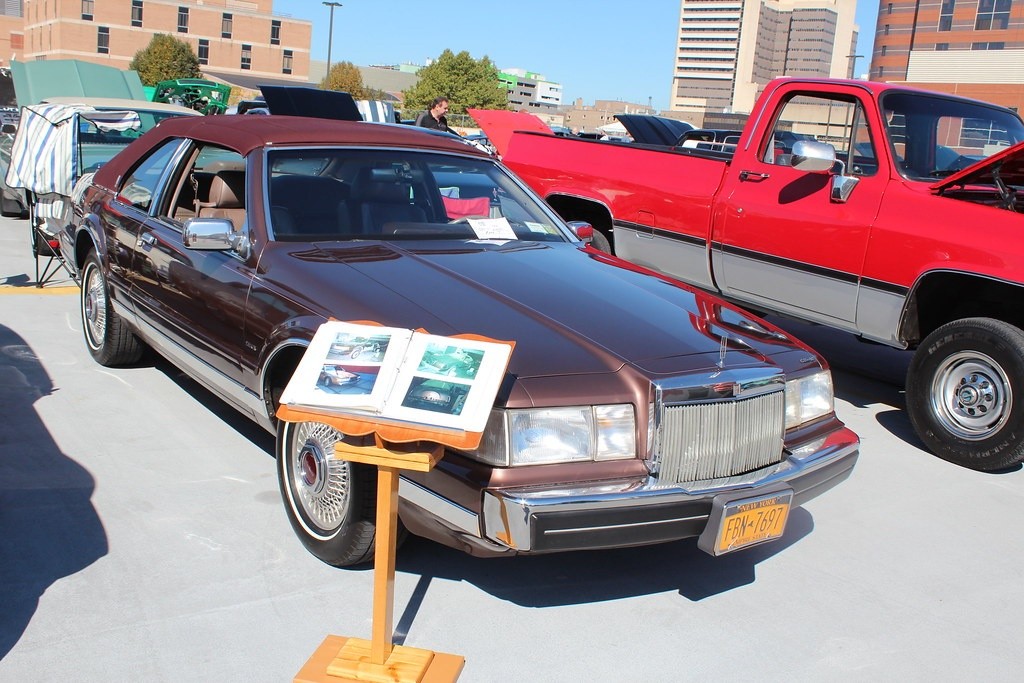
[[0, 58, 989, 257], [331, 334, 389, 359], [318, 363, 361, 387], [55, 113, 862, 574]]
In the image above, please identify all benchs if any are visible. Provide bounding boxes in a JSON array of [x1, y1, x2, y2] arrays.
[[168, 174, 348, 238]]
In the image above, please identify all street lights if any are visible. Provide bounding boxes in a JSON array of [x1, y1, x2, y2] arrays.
[[322, 0, 344, 89]]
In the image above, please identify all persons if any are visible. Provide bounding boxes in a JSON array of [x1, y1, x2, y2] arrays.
[[621, 130, 631, 143], [579, 125, 585, 133], [413, 97, 448, 132], [600, 130, 608, 141]]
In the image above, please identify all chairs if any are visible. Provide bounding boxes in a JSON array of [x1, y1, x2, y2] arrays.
[[200, 168, 248, 234], [340, 169, 431, 236]]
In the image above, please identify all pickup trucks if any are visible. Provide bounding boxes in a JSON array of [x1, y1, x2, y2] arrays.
[[499, 75, 1024, 473]]
